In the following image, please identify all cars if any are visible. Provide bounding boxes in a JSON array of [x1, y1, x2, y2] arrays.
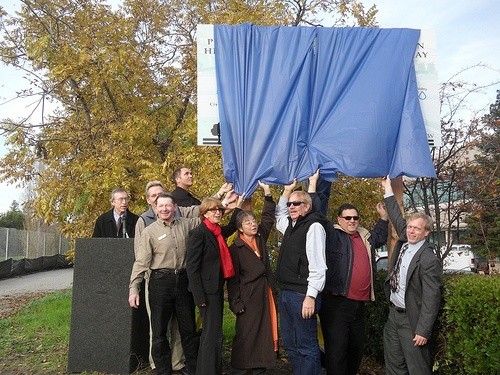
[[374, 251, 389, 272], [433, 245, 475, 274]]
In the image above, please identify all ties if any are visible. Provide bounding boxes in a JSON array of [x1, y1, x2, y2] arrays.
[[116, 216, 123, 238]]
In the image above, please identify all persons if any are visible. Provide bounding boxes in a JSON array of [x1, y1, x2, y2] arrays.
[[381, 175, 440, 375], [228, 180, 278, 375], [92, 188, 141, 238], [308, 169, 389, 375], [129, 167, 245, 375], [275, 178, 328, 375]]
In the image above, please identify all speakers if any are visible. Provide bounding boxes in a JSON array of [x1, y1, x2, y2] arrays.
[[67, 237, 150, 375]]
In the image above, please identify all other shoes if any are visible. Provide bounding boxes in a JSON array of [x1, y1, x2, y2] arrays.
[[153, 368, 160, 375], [178, 366, 193, 375], [160, 369, 173, 375], [232, 366, 267, 375]]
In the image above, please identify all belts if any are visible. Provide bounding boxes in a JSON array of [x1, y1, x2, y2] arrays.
[[154, 268, 187, 274], [389, 301, 407, 313]]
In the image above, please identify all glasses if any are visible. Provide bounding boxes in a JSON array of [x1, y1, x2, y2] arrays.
[[287, 202, 307, 207], [242, 219, 258, 225], [114, 197, 131, 202], [341, 216, 360, 220], [206, 207, 223, 213]]
